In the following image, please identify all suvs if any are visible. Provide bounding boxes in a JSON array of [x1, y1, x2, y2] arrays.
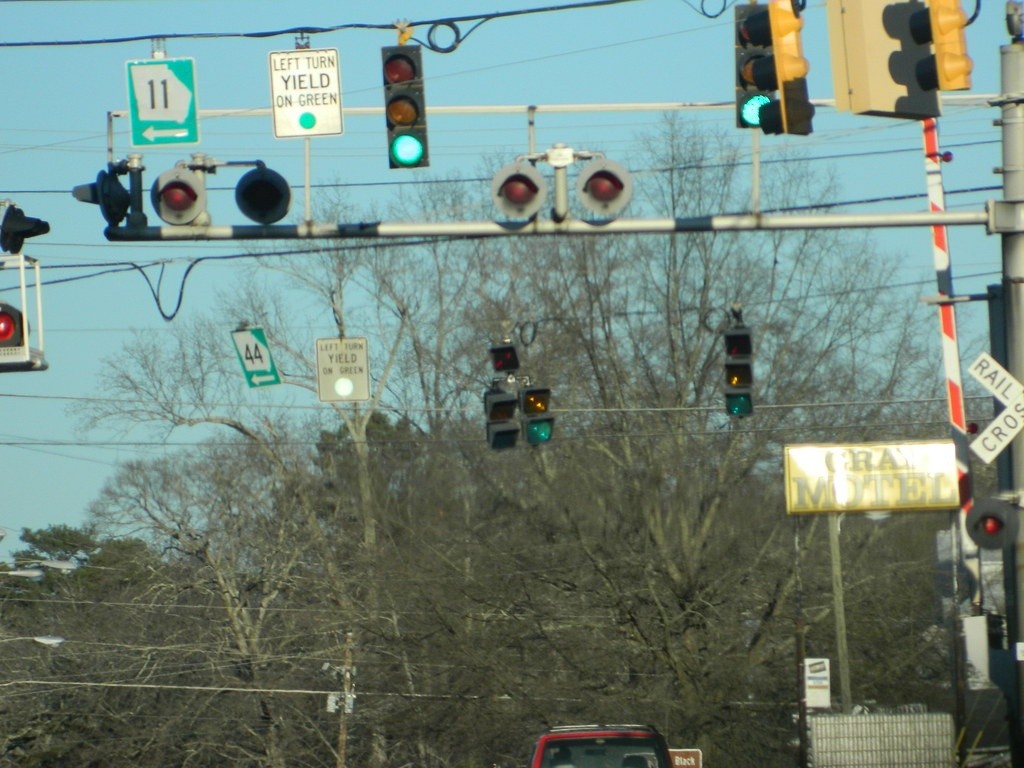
[[529, 723, 673, 768]]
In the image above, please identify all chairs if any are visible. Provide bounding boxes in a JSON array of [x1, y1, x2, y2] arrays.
[[621, 756, 648, 768]]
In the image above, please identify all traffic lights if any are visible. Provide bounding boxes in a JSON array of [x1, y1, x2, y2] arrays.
[[0, 198, 50, 255], [0, 300, 31, 348], [966, 498, 1017, 552], [487, 150, 630, 220], [381, 44, 428, 169], [150, 154, 290, 225], [909, 0, 973, 92], [747, 0, 815, 135], [70, 170, 128, 228], [487, 340, 554, 451], [733, 4, 777, 128], [724, 327, 753, 418]]
